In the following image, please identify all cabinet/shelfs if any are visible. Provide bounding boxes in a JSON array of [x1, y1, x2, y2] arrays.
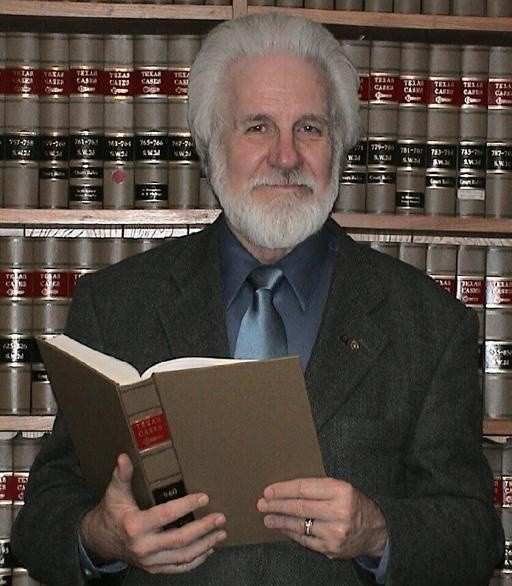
[[0, 0, 512, 437]]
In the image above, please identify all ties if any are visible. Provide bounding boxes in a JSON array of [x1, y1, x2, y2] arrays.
[[234, 264, 287, 361]]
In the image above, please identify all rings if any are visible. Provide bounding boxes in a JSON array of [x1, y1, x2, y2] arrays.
[[304, 518, 317, 535]]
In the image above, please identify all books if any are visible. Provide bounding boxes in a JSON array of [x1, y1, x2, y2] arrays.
[[479, 441, 501, 585], [361, 242, 512, 417], [1, 439, 15, 570], [11, 432, 47, 526], [337, 40, 511, 219], [35, 332, 329, 549], [0, 31, 221, 208], [0, 237, 181, 415], [501, 444, 511, 586]]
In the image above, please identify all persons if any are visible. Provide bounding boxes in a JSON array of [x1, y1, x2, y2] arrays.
[[9, 0, 507, 586]]
